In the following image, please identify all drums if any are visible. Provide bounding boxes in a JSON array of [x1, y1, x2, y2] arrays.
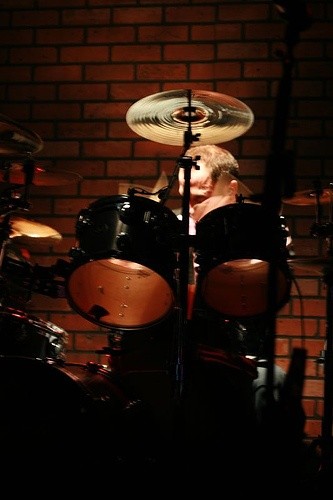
[[193, 202, 293, 323], [0, 304, 68, 380], [64, 194, 180, 330], [14, 357, 137, 480]]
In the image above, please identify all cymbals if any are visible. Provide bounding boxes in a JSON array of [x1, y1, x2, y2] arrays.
[[2, 215, 62, 239], [1, 159, 83, 187], [1, 118, 44, 156], [280, 187, 333, 206], [126, 87, 254, 148]]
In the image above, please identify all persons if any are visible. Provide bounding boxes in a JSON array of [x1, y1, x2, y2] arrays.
[[164, 143, 304, 500]]
